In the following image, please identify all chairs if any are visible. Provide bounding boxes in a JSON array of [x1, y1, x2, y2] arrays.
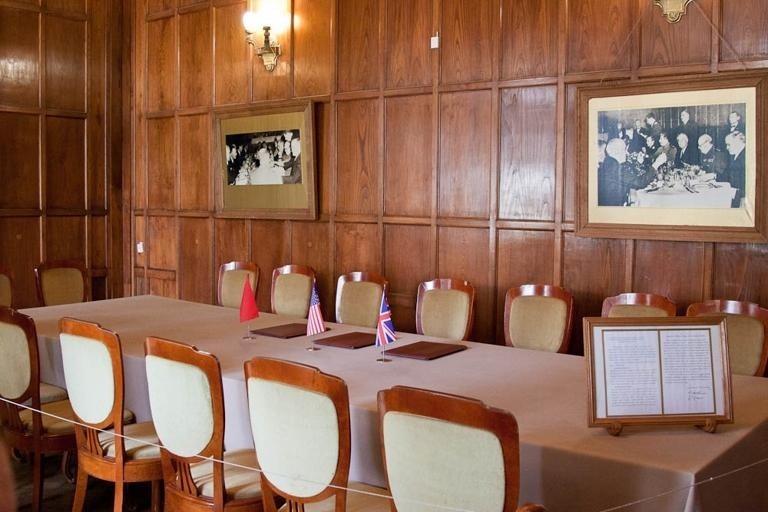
[[1, 259, 766, 511]]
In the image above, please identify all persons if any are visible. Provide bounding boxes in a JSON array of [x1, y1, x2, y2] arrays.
[[224, 129, 301, 186], [596, 106, 745, 207]]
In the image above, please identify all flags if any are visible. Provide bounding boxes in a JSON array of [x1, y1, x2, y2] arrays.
[[239, 275, 260, 324], [376, 286, 400, 349], [304, 284, 327, 338]]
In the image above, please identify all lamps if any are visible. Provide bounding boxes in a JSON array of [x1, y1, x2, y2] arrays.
[[241, 11, 284, 73]]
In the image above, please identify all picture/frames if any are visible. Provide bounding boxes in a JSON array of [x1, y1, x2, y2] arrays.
[[206, 97, 320, 222], [573, 66, 768, 246]]
